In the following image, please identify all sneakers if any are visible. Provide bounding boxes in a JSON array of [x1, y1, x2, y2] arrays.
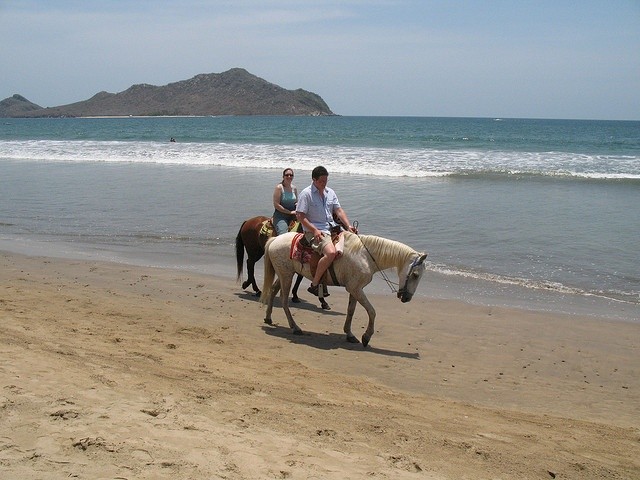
[[308, 283, 330, 297]]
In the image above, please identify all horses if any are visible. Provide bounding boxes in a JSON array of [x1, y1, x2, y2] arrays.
[[234, 215, 332, 310], [258, 230, 428, 348]]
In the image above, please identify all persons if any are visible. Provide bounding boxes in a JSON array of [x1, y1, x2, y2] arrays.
[[272, 169, 299, 236], [296, 166, 358, 298]]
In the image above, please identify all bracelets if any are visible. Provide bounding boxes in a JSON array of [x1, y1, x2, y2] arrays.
[[345, 225, 351, 230]]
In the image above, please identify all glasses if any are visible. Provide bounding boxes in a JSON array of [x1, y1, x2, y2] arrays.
[[284, 175, 293, 176]]
